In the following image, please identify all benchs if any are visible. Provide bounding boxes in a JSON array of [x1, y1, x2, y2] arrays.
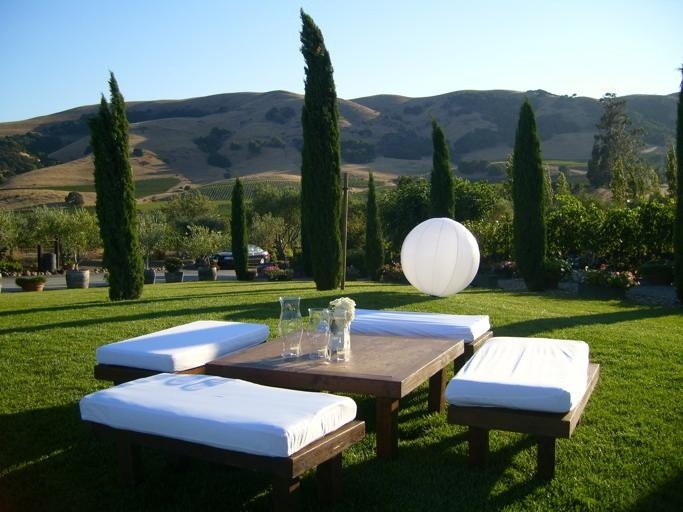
[[445, 337, 601, 479], [93, 320, 271, 383], [79, 370, 366, 511], [320, 310, 494, 375]]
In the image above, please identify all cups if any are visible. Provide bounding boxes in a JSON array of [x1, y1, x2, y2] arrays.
[[329, 310, 346, 361], [308, 308, 329, 360]]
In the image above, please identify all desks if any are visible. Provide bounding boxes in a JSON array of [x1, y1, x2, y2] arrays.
[[205, 333, 465, 461]]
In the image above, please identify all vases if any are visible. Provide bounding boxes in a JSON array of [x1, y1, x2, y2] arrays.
[[329, 296, 356, 353], [307, 308, 331, 359]]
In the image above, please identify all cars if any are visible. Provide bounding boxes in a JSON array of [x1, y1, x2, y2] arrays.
[[197, 244, 270, 269]]
